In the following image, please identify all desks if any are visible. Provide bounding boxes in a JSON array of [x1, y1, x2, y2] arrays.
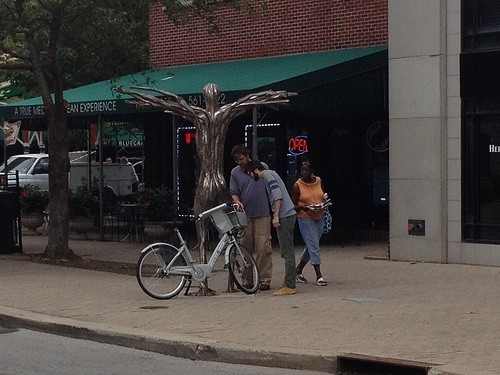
[[121, 203, 149, 244]]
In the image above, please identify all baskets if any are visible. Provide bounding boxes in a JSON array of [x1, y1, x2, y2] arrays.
[[211, 211, 247, 233]]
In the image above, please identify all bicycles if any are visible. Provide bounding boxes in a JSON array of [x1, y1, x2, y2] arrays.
[[136, 200, 260, 300]]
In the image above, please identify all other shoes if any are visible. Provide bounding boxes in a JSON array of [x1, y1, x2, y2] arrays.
[[316, 278, 328, 286], [260, 285, 270, 290], [273, 287, 296, 295], [296, 274, 307, 283]]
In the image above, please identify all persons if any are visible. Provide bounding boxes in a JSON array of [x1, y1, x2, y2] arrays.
[[245, 160, 297, 295], [114, 82, 298, 295], [229, 145, 273, 290], [291, 162, 328, 286]]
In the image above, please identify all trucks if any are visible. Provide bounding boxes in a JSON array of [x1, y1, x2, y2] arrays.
[[0, 151, 139, 213]]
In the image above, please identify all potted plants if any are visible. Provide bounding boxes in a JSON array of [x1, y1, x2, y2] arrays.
[[21, 212, 43, 236], [69, 177, 99, 233], [138, 184, 173, 240]]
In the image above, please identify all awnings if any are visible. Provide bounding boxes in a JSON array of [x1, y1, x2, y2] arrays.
[[0, 45, 388, 243]]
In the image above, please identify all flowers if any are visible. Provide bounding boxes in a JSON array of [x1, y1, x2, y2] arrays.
[[19, 184, 49, 213]]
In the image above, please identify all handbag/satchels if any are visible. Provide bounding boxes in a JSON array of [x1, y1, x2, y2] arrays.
[[323, 200, 332, 234]]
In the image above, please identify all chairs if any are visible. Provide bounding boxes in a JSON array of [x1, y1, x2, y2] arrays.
[[103, 195, 132, 241]]
